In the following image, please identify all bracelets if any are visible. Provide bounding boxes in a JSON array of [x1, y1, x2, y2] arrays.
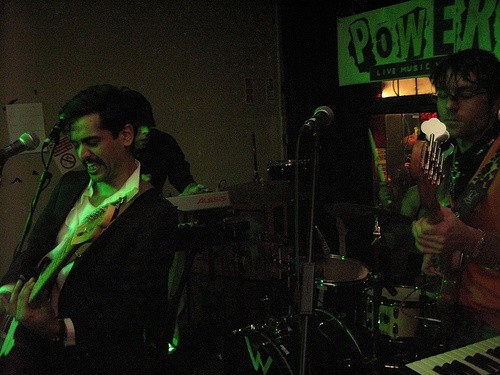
[[42, 321, 65, 343], [470, 229, 488, 259]]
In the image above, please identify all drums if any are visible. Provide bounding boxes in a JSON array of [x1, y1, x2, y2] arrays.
[[268, 158, 320, 201], [220, 308, 371, 375], [358, 285, 421, 343], [281, 253, 370, 333]]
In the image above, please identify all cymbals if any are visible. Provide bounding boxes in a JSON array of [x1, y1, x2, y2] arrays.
[[341, 200, 413, 225]]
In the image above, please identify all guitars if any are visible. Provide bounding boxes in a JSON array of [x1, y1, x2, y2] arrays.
[[0, 205, 119, 375], [407, 136, 465, 300]]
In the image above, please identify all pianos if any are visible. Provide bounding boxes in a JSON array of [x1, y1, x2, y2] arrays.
[[172, 219, 251, 300], [164, 180, 273, 211], [400, 335, 500, 375]]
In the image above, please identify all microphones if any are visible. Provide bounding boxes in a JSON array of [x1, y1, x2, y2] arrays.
[[304, 106, 334, 132], [379, 275, 397, 296], [315, 224, 330, 254], [42, 113, 69, 149], [0, 131, 40, 162]]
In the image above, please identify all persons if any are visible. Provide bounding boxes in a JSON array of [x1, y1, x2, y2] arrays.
[[0, 84, 179, 375], [378, 179, 450, 246], [127, 90, 210, 315], [412, 47, 500, 348]]
